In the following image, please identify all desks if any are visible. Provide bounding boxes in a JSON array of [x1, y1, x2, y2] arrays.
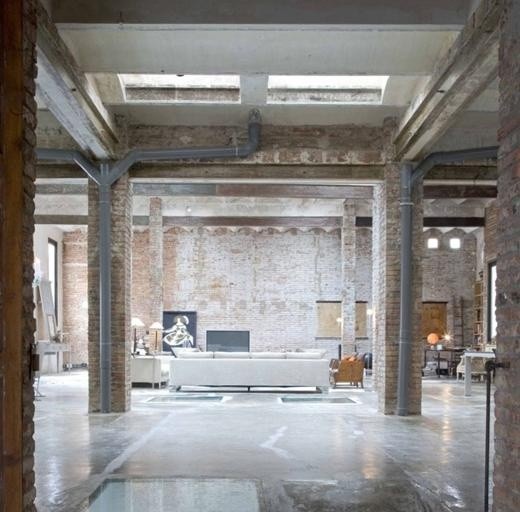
[[422, 345, 479, 379]]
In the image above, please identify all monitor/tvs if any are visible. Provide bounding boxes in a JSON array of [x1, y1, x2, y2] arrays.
[[206, 330, 250, 352]]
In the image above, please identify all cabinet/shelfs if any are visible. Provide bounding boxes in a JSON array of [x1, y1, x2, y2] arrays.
[[472, 280, 483, 344]]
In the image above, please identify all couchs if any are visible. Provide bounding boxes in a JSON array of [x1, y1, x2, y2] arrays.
[[170, 351, 331, 392]]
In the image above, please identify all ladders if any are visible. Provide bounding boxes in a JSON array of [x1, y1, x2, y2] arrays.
[[452, 295, 464, 375], [472, 276, 485, 350]]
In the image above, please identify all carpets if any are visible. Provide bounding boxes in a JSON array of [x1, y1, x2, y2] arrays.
[[148, 395, 223, 403], [279, 397, 356, 404]]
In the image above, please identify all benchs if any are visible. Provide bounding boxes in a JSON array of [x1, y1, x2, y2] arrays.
[[457, 352, 496, 381]]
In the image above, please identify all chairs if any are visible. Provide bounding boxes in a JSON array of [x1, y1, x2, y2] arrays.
[[332, 352, 365, 389], [129, 351, 174, 389]]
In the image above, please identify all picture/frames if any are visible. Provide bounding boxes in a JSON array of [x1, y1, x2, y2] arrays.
[[163, 311, 197, 352]]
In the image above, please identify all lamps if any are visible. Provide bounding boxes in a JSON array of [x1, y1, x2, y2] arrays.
[[130, 317, 164, 358]]
